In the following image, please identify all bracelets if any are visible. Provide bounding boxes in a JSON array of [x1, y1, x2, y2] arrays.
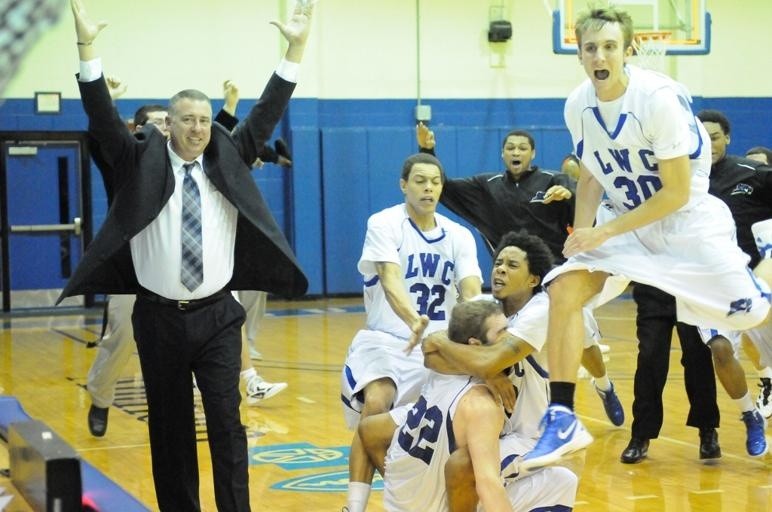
[[76, 41, 93, 47]]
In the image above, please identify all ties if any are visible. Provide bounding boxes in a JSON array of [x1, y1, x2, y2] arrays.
[[181, 164, 203, 292]]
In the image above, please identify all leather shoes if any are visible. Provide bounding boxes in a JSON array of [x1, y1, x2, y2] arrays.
[[88, 404, 108, 436]]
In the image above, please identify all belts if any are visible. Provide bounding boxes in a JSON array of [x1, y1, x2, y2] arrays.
[[140, 289, 230, 311]]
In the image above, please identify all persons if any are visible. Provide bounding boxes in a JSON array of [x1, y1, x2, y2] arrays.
[[337, 107, 771, 512], [211, 80, 295, 410], [83, 72, 173, 439], [499, 2, 770, 477], [68, 0, 319, 512]]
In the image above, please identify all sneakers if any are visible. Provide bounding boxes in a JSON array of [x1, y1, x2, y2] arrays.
[[621, 438, 649, 462], [524, 408, 594, 470], [699, 429, 720, 457], [593, 379, 624, 426], [246, 382, 287, 403], [743, 378, 772, 455]]
